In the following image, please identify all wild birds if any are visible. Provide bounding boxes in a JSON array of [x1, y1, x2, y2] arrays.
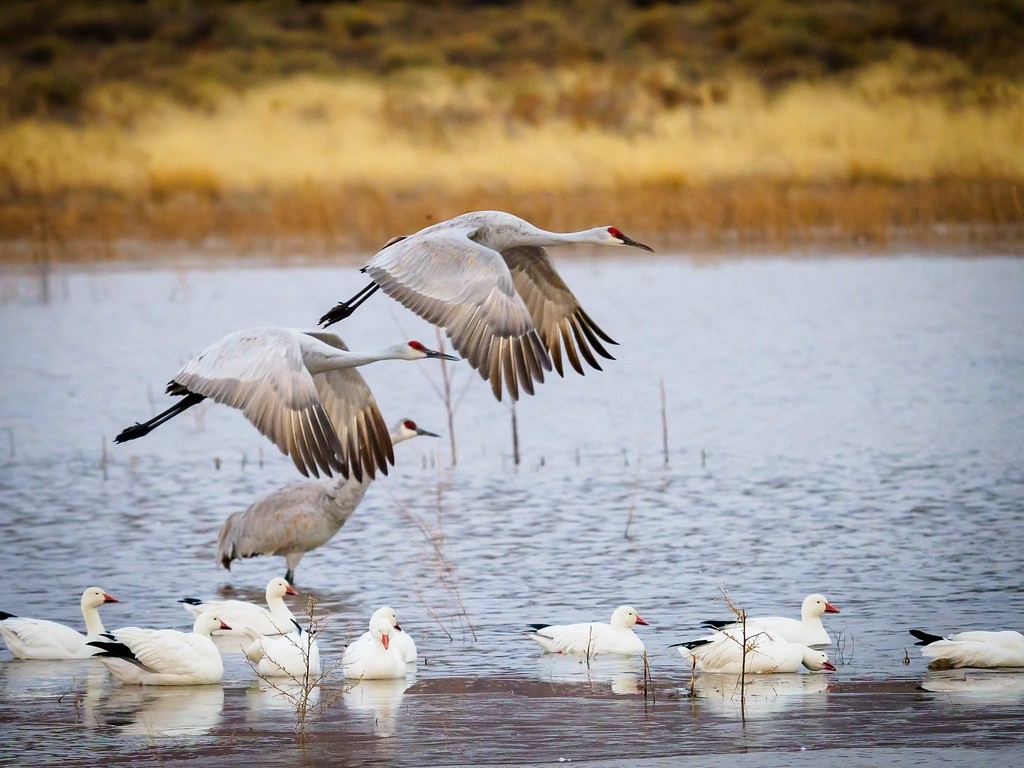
[[112, 326, 461, 484], [317, 210, 656, 403], [0, 586, 120, 662], [369, 606, 418, 663], [177, 575, 299, 635], [214, 416, 442, 585], [667, 631, 836, 675], [85, 611, 232, 687], [241, 617, 320, 676], [342, 617, 407, 679], [699, 593, 840, 647], [523, 604, 650, 656], [908, 629, 1024, 668]]
